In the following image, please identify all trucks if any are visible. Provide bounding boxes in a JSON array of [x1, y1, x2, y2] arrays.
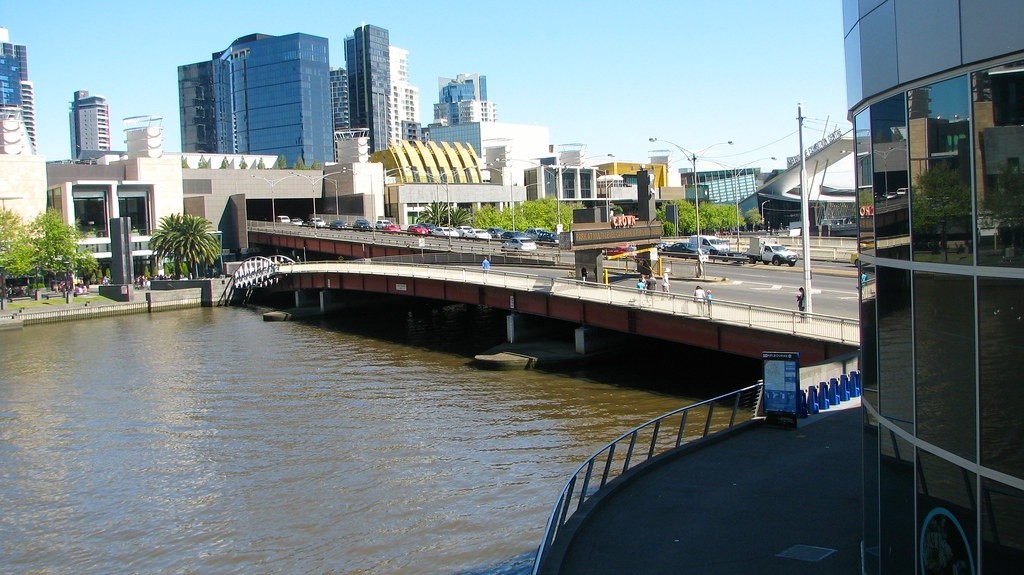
[[742, 237, 799, 267], [690, 235, 730, 255]]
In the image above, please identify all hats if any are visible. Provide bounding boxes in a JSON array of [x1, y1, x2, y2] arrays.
[[664, 267, 671, 273]]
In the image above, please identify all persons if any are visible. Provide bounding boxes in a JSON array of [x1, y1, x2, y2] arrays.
[[481, 257, 490, 274], [581, 265, 588, 280], [636, 273, 657, 307], [796, 286, 804, 322], [6, 284, 13, 302], [136, 275, 148, 289], [103, 274, 110, 285], [693, 285, 712, 314], [75, 285, 88, 295], [57, 281, 63, 291], [661, 268, 671, 301], [151, 273, 175, 280], [861, 270, 867, 287]]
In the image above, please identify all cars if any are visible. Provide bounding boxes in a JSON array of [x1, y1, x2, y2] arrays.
[[329, 220, 347, 230], [376, 220, 400, 234], [407, 223, 560, 253], [291, 217, 327, 228], [604, 246, 634, 255], [657, 241, 710, 259], [874, 187, 908, 203], [352, 219, 373, 232]]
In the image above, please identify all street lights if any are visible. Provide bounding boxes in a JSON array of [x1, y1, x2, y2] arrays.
[[342, 157, 635, 232], [649, 138, 734, 277], [692, 157, 778, 253], [873, 147, 906, 207], [289, 171, 345, 228], [251, 174, 312, 232], [762, 200, 770, 230]]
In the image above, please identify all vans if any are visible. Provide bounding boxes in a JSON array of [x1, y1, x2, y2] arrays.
[[276, 215, 290, 223]]
